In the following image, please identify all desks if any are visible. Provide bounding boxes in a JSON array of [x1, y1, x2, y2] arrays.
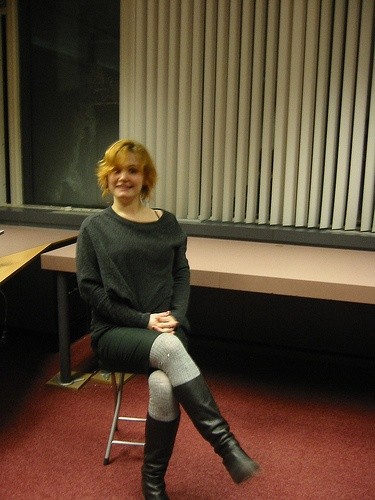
[[41, 236, 375, 382], [0, 224, 81, 383]]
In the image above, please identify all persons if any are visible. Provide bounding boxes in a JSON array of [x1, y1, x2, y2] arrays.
[[76, 138, 260, 500]]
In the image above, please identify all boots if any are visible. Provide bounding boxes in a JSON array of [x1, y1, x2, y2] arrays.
[[172, 373, 259, 484], [141, 410, 181, 500]]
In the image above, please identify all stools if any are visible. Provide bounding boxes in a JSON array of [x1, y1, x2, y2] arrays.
[[103, 372, 146, 465]]
